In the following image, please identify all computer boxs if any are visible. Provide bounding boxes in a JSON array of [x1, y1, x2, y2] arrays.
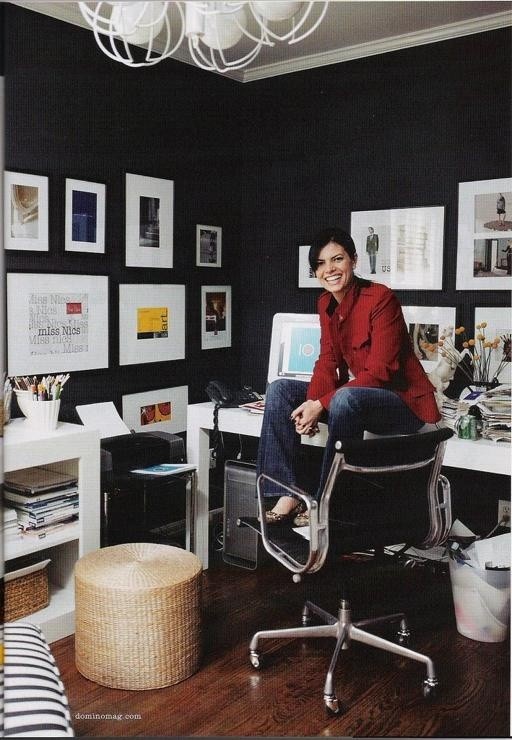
[[222, 456, 258, 571]]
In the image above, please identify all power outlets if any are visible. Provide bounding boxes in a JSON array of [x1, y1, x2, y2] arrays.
[[496, 498, 511, 529]]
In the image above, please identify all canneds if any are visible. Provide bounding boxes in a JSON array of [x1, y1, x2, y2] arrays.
[[458, 415, 477, 440]]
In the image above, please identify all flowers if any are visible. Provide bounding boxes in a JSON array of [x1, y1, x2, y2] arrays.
[[424, 321, 511, 391]]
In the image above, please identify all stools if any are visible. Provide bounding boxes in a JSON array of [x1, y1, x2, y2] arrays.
[[0, 624, 75, 738], [71, 540, 208, 696]]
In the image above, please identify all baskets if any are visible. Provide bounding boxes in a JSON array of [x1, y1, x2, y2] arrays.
[[0, 563, 49, 624]]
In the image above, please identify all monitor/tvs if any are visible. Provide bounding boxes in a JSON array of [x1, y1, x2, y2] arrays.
[[268, 313, 339, 382]]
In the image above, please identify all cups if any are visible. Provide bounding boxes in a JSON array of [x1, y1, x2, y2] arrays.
[[2, 388, 14, 425], [34, 399, 61, 431]]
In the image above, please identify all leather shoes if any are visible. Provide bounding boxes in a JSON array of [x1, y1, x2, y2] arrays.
[[257, 501, 306, 525], [294, 512, 311, 525]]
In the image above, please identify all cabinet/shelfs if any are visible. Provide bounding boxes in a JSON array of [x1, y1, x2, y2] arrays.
[[1, 417, 102, 646]]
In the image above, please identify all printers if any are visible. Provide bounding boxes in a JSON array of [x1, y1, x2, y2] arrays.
[[100, 430, 186, 550]]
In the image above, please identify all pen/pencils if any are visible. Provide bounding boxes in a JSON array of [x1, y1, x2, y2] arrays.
[[13, 373, 70, 401]]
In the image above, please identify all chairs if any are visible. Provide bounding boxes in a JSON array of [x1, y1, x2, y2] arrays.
[[234, 424, 458, 719]]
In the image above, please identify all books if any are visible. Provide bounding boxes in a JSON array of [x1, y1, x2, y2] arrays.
[[239, 399, 265, 414], [440, 381, 511, 444], [1, 467, 79, 541]]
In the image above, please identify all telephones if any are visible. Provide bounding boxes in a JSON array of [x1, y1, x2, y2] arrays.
[[206, 381, 264, 407]]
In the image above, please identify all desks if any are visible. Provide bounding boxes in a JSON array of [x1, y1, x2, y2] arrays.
[[100, 461, 199, 555], [184, 392, 511, 574]]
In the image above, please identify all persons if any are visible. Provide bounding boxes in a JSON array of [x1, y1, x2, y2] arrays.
[[366, 226, 379, 274], [501, 239, 512, 276], [253, 227, 441, 529], [496, 193, 507, 228], [207, 292, 225, 331]]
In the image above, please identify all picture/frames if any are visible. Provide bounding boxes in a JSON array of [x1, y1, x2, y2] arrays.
[[399, 304, 458, 378], [298, 245, 323, 291], [121, 385, 189, 438], [196, 223, 223, 269], [453, 177, 511, 293], [198, 285, 234, 352], [470, 304, 511, 387], [350, 205, 446, 294], [3, 165, 186, 382]]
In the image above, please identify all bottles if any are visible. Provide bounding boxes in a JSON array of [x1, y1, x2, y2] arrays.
[[459, 415, 478, 441]]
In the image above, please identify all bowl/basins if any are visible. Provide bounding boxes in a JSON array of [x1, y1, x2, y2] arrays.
[[15, 389, 36, 424]]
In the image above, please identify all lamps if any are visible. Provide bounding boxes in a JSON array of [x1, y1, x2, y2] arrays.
[[75, 1, 331, 78]]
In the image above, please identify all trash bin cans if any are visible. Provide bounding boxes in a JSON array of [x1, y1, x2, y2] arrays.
[[448, 556, 511, 643]]
